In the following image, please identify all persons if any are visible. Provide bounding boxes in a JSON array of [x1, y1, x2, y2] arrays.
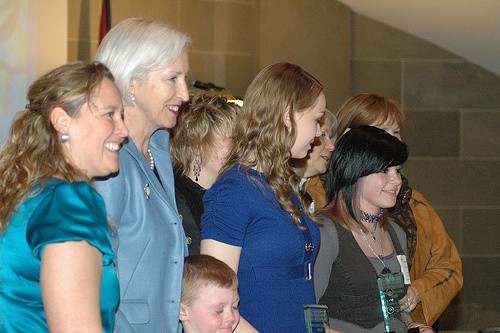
[[0, 59, 130, 333], [169, 88, 241, 255], [292, 107, 339, 219], [179, 254, 240, 333], [311, 125, 436, 333], [305, 93, 463, 333], [92, 17, 190, 333], [200, 62, 327, 333]]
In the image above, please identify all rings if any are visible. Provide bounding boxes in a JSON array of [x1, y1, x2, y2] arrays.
[[406, 298, 413, 308]]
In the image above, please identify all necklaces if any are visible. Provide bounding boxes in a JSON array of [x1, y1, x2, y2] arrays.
[[148, 149, 154, 170], [359, 229, 387, 267], [359, 208, 383, 223], [366, 223, 377, 241]]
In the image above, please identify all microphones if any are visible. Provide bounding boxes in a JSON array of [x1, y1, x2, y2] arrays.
[[188, 80, 226, 93]]
